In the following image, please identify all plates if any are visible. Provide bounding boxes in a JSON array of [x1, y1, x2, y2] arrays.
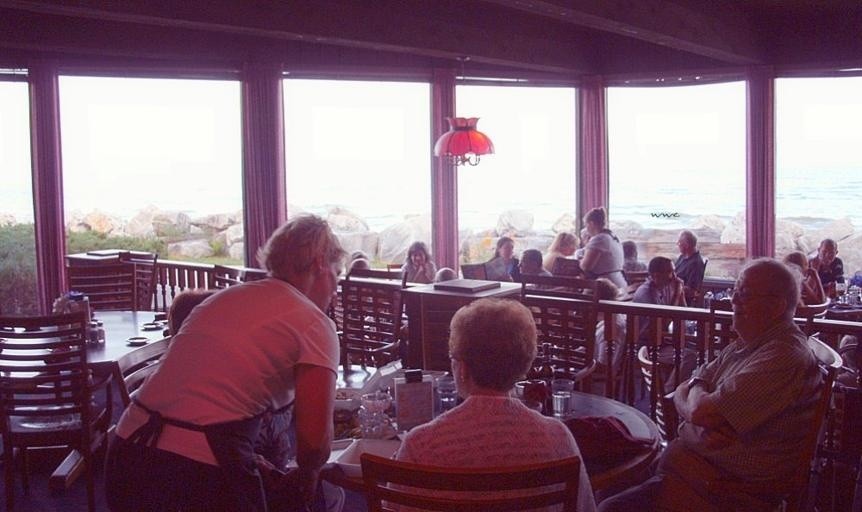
[[126, 318, 171, 346]]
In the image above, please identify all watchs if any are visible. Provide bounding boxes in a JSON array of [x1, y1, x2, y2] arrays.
[[687, 377, 710, 394]]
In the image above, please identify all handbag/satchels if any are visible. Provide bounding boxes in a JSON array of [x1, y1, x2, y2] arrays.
[[564, 416, 657, 460]]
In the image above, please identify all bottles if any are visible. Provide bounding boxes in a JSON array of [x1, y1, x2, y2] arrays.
[[63, 291, 107, 347]]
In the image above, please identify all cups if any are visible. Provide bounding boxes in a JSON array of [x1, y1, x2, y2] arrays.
[[551, 379, 575, 392], [833, 280, 856, 306], [706, 286, 732, 305], [550, 391, 571, 415], [362, 371, 460, 440]]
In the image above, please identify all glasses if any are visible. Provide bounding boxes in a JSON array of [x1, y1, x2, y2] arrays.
[[727, 287, 780, 300]]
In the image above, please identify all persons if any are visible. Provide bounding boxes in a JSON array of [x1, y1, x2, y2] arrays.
[[103, 214, 348, 512], [484, 229, 647, 298], [345, 251, 458, 284], [383, 296, 596, 512], [675, 231, 705, 298], [574, 278, 647, 405], [626, 257, 687, 347], [578, 207, 629, 297], [782, 239, 862, 312], [401, 242, 437, 284], [166, 289, 345, 511], [596, 256, 825, 512]]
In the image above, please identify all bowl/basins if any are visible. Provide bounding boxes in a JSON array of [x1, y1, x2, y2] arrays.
[[514, 377, 546, 408], [331, 388, 361, 412]]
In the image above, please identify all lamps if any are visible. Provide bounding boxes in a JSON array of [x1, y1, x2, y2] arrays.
[[433, 56, 495, 166]]
